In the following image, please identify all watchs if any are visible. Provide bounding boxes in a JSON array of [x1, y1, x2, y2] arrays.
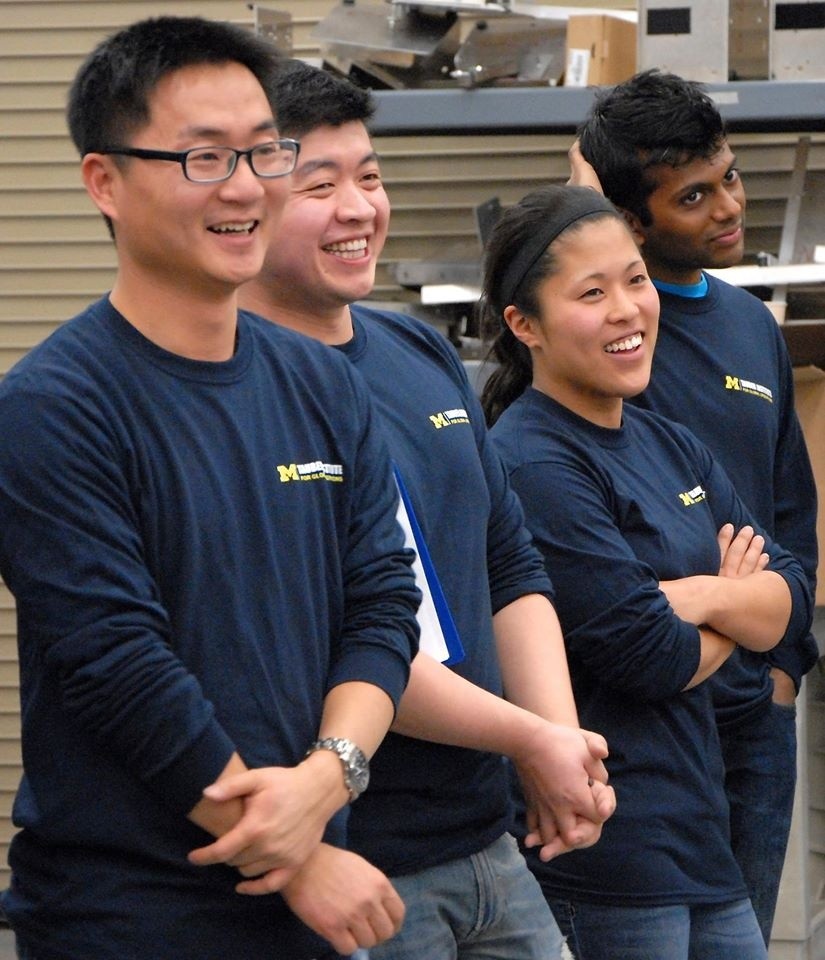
[[303, 736, 370, 803]]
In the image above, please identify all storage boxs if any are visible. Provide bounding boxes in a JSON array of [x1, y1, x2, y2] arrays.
[[563, 10, 638, 92]]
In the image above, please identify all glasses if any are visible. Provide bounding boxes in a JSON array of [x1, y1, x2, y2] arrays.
[[93, 136, 301, 183]]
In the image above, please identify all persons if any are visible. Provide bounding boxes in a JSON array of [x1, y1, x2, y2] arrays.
[[563, 69, 817, 952], [0, 14, 423, 960], [454, 184, 814, 960], [233, 55, 618, 960]]
